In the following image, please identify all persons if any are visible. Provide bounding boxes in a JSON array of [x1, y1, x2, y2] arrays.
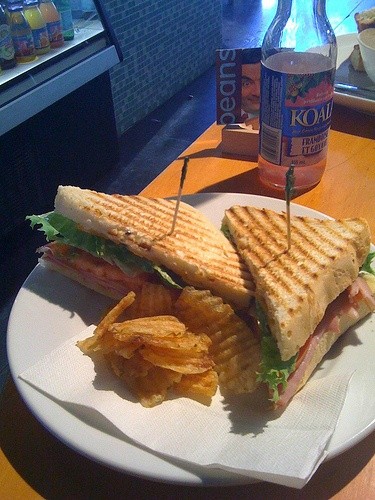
[[241, 48, 262, 123]]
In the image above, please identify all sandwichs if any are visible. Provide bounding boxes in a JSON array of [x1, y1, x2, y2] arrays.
[[25, 186, 255, 304], [224, 205, 375, 410]]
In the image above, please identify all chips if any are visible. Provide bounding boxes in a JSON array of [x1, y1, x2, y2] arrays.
[[77, 287, 267, 406]]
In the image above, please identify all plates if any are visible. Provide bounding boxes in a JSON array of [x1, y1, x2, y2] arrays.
[[304, 33, 375, 117], [6, 193, 375, 488]]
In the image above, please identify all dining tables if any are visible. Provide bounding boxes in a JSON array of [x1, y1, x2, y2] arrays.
[[0, 99, 375, 500]]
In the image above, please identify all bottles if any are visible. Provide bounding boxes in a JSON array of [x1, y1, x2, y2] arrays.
[[258, 0, 338, 193], [0, 0, 75, 70]]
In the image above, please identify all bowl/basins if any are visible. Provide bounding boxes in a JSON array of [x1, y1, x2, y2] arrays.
[[357, 27, 375, 85]]
